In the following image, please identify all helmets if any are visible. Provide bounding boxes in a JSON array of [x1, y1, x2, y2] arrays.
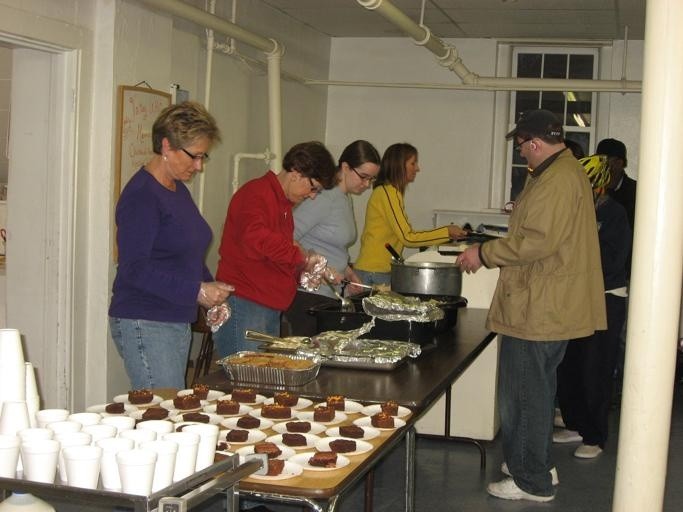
[[578, 154, 611, 190]]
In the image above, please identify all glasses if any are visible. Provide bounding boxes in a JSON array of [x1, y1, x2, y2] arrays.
[[180, 147, 209, 164], [309, 176, 322, 195], [350, 165, 377, 184]]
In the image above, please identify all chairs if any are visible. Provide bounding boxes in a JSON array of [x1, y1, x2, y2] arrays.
[[83, 390, 416, 512]]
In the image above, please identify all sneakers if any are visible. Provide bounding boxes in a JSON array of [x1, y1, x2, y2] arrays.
[[487, 407, 604, 503]]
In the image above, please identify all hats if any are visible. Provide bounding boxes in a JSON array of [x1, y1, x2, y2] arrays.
[[596, 139, 626, 159], [505, 110, 563, 139]]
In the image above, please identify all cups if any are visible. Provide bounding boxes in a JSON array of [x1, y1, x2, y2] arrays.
[[0, 327, 220, 497]]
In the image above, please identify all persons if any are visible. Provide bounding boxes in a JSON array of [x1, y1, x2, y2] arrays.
[[350, 141, 467, 299], [207, 141, 342, 375], [592, 137, 638, 425], [453, 106, 608, 500], [284, 139, 382, 338], [550, 154, 628, 461], [106, 101, 235, 399]]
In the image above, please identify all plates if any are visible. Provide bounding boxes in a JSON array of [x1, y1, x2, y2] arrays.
[[462, 229, 504, 242]]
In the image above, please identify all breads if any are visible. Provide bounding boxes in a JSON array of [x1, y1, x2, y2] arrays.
[[227, 353, 314, 370], [106, 385, 397, 475]]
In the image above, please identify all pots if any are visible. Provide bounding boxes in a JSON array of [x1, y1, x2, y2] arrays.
[[385, 242, 463, 296]]
